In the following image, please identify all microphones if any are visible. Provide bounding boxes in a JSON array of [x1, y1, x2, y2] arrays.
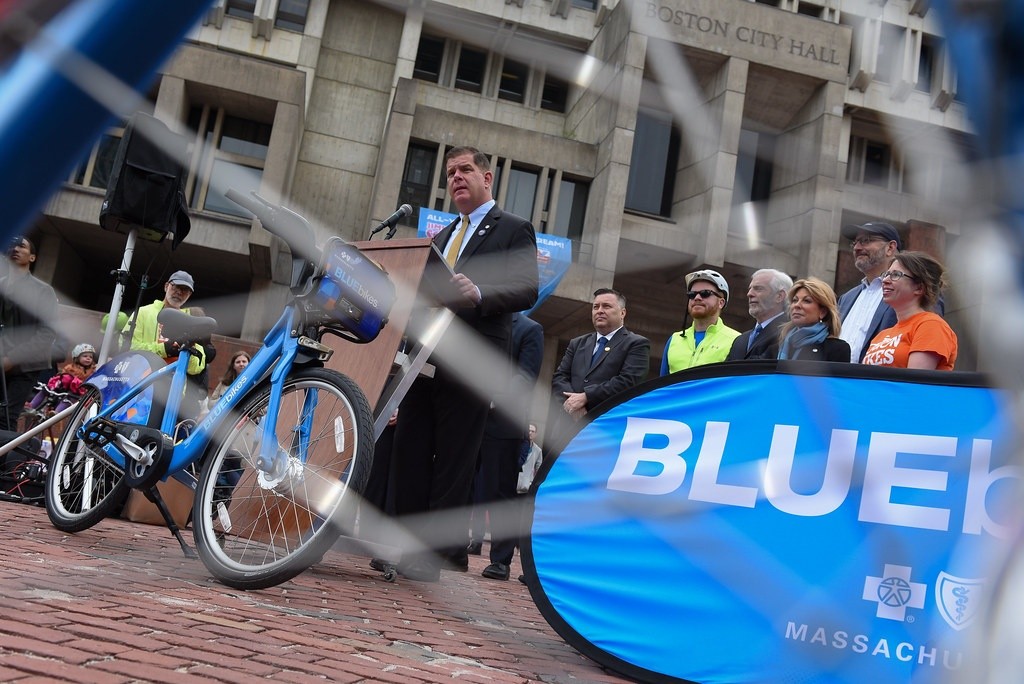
[[373, 203, 412, 234]]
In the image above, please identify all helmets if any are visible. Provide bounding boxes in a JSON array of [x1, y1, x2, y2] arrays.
[[685, 270, 730, 308], [72, 343, 96, 359]]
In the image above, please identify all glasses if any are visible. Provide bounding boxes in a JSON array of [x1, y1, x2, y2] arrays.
[[850, 235, 887, 249], [170, 284, 191, 294], [882, 270, 913, 282], [687, 289, 722, 299]]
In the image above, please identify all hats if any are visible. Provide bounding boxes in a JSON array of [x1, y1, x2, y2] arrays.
[[843, 220, 901, 251], [168, 271, 194, 292]]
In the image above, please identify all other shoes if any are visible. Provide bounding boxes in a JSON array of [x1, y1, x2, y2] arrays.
[[49, 410, 58, 418], [369, 558, 402, 576], [403, 558, 441, 583], [467, 540, 483, 555]]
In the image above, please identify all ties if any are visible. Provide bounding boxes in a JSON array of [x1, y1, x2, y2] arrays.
[[746, 324, 763, 352], [445, 215, 469, 270], [590, 337, 608, 367]]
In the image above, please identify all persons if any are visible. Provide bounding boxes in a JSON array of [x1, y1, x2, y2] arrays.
[[723, 269, 793, 360], [371, 144, 538, 581], [365, 310, 543, 580], [660, 268, 741, 377], [861, 251, 958, 371], [777, 279, 851, 364], [553, 287, 650, 452], [835, 222, 945, 363], [0, 237, 266, 521]]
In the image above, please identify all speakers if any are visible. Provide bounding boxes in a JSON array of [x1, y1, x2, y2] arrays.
[[99, 111, 189, 243]]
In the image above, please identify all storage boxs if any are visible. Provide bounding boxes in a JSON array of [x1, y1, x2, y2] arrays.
[[123, 472, 200, 527]]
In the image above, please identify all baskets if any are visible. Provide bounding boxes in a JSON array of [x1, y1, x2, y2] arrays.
[[298, 236, 398, 342]]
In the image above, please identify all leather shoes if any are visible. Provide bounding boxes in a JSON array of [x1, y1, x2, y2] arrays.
[[482, 562, 511, 581], [442, 554, 469, 572]]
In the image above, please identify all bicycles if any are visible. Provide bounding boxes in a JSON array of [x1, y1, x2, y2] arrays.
[[40, 183, 397, 593], [0, 375, 85, 507]]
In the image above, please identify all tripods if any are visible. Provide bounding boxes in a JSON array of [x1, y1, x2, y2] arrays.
[[0, 231, 138, 515]]
